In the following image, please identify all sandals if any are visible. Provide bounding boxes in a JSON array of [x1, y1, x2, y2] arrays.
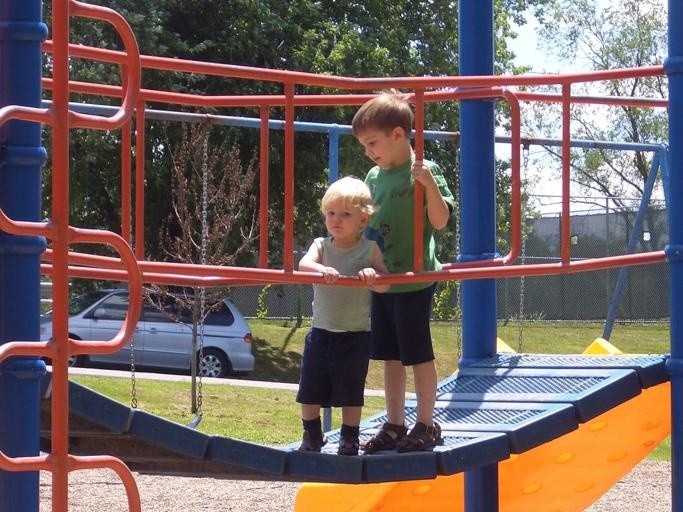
[[364, 422, 444, 453]]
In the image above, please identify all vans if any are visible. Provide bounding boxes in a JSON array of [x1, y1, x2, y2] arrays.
[[39, 288, 255, 377]]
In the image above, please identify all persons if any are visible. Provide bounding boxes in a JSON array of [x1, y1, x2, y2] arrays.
[[351, 95, 455, 453], [296, 176, 390, 456]]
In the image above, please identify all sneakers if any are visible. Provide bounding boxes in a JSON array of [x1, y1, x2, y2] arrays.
[[337, 433, 359, 455], [295, 430, 328, 452]]
